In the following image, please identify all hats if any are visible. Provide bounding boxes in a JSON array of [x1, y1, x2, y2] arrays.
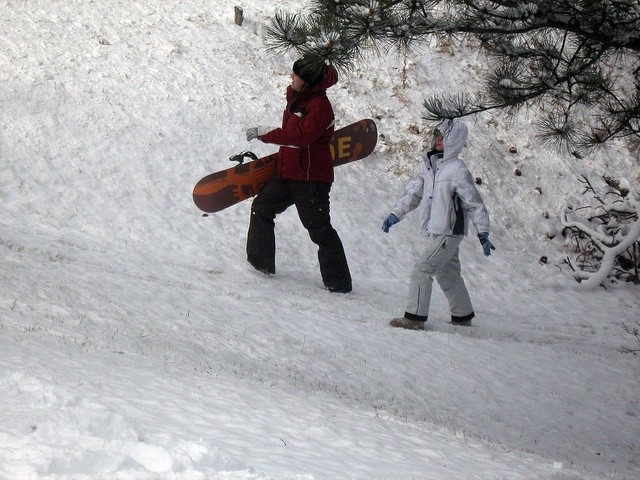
[[292, 52, 327, 86]]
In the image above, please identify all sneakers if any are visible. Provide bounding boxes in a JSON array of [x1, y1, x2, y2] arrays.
[[389, 317, 425, 330], [449, 319, 472, 327], [241, 261, 275, 279], [312, 278, 354, 297]]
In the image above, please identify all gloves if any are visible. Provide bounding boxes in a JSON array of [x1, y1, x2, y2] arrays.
[[245, 128, 258, 141], [477, 232, 495, 257], [381, 214, 400, 233]]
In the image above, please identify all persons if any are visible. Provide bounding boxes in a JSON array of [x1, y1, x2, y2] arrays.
[[247, 54, 353, 294], [380, 122, 495, 331]]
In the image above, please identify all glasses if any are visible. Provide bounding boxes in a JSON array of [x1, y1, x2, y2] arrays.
[[434, 132, 444, 141]]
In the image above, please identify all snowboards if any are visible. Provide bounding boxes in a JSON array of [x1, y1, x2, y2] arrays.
[[192, 116, 378, 214]]
